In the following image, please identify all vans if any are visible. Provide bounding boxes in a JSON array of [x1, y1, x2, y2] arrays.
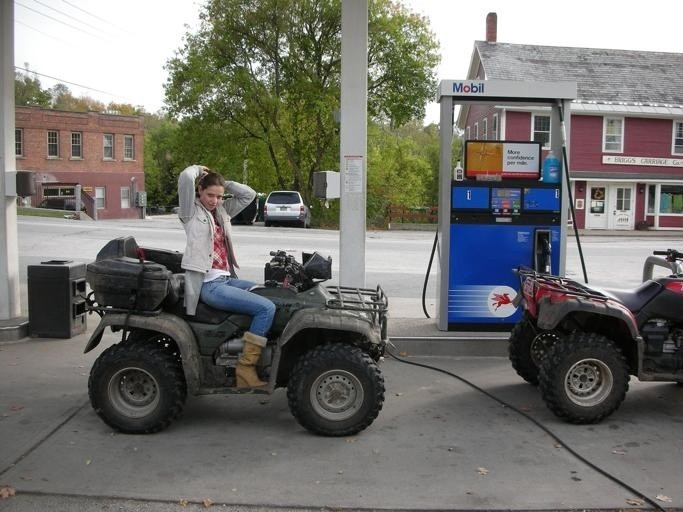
[[263, 190, 313, 228], [37, 198, 87, 213]]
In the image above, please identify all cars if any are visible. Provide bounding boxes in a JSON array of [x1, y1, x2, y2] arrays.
[[151, 205, 179, 213]]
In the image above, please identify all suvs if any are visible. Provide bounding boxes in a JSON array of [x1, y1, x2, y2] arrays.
[[506, 249, 683, 426], [76, 235, 389, 437]]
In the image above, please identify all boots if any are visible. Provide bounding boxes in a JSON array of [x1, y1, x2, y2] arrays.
[[233, 331, 270, 390]]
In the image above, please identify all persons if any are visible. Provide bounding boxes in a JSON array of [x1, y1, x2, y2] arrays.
[[177, 163, 275, 392]]
